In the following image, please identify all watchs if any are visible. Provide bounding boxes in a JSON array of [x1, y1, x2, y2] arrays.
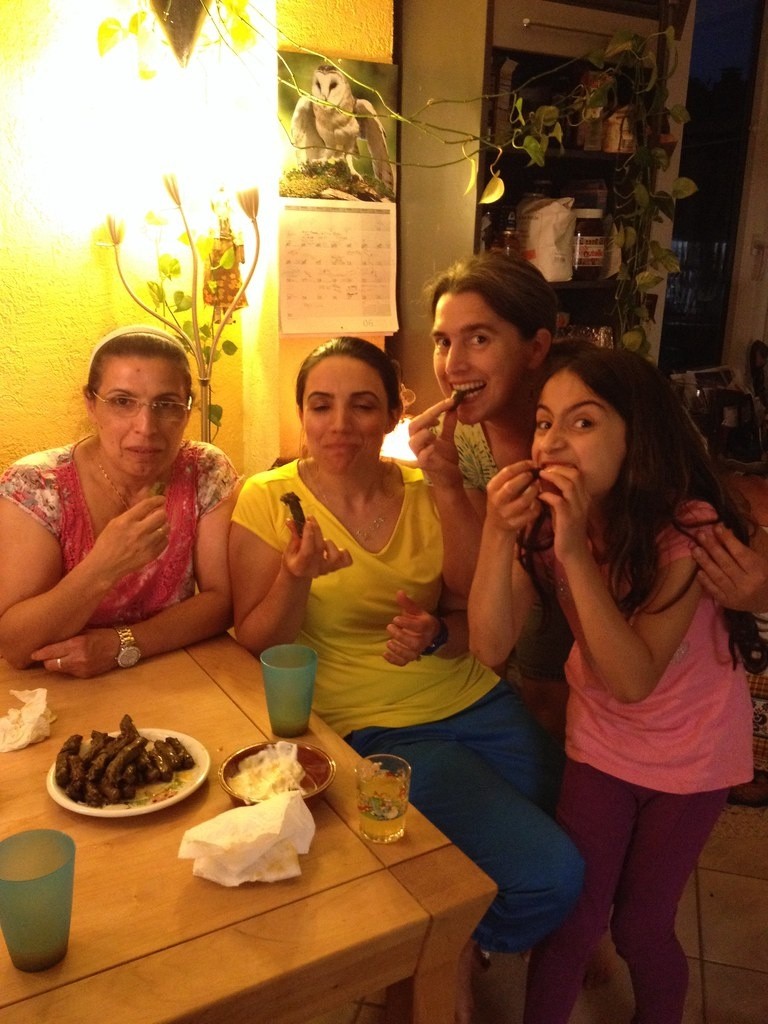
[[423, 613, 448, 657], [114, 624, 140, 668]]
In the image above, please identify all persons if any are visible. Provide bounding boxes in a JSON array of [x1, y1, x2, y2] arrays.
[[409, 253, 768, 741], [229, 336, 587, 1023], [468, 346, 754, 1024], [0, 325, 241, 679]]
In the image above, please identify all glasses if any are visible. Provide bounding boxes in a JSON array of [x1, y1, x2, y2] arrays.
[[92, 389, 192, 426]]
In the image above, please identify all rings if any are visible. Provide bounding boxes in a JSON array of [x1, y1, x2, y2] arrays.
[[57, 659, 61, 669], [157, 529, 165, 537]]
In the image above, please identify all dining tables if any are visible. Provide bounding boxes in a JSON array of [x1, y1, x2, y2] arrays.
[[0, 632, 498, 1024]]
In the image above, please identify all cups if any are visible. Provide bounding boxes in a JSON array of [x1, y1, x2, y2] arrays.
[[259, 643, 318, 738], [354, 755, 412, 844], [0, 828, 75, 973]]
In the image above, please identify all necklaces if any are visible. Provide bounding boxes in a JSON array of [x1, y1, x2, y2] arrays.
[[322, 493, 384, 541], [99, 464, 129, 510]]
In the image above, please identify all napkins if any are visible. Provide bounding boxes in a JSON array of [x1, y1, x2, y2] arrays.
[[176, 790, 317, 887], [0, 688, 57, 754]]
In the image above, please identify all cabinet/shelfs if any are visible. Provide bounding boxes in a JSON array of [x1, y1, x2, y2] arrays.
[[396, 0, 698, 414]]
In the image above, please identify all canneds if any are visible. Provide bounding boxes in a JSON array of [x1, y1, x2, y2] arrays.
[[487, 178, 605, 280]]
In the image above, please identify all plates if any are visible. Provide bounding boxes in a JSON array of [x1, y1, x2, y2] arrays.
[[45, 728, 209, 818]]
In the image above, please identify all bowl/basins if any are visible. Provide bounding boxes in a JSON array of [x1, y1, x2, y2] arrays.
[[216, 739, 336, 808]]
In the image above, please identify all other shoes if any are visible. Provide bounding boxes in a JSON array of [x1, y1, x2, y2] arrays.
[[523, 947, 614, 992]]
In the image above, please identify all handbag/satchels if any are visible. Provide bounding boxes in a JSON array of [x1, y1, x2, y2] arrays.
[[514, 196, 575, 283]]
